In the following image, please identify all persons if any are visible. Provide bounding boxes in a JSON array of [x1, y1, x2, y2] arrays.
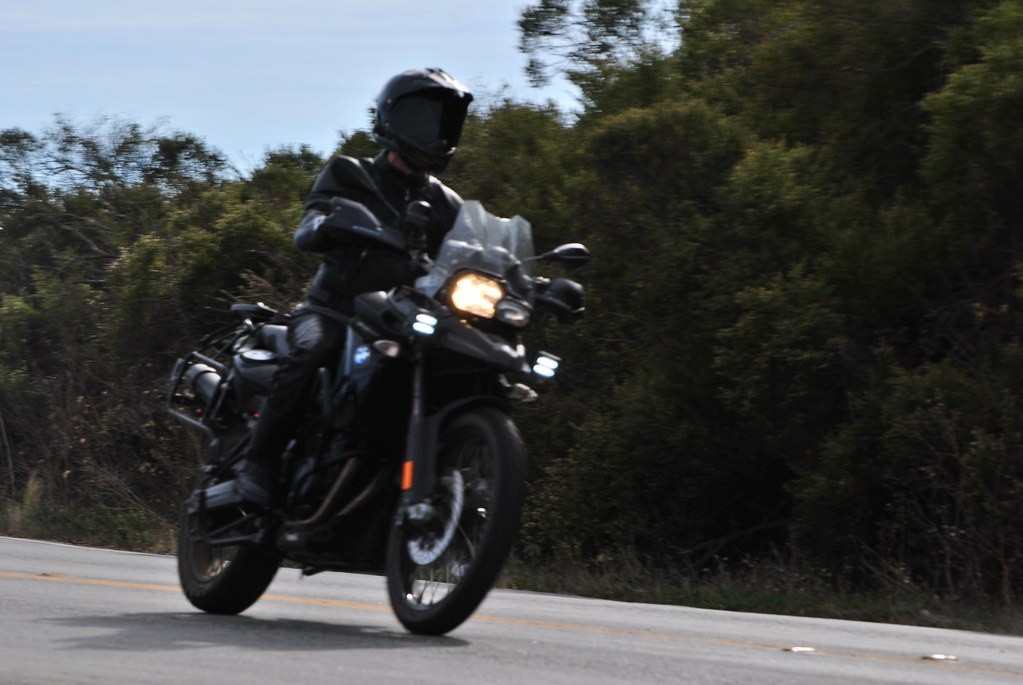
[[237, 65, 586, 510]]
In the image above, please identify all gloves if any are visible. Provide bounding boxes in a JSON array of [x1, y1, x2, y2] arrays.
[[548, 278, 585, 326], [328, 196, 381, 243]]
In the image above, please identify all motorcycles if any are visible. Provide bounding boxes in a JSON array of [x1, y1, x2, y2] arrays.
[[162, 154, 594, 636]]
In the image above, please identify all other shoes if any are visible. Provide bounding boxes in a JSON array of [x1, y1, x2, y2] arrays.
[[233, 447, 286, 508]]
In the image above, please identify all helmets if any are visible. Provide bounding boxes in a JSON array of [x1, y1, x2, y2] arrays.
[[372, 67, 473, 174]]
